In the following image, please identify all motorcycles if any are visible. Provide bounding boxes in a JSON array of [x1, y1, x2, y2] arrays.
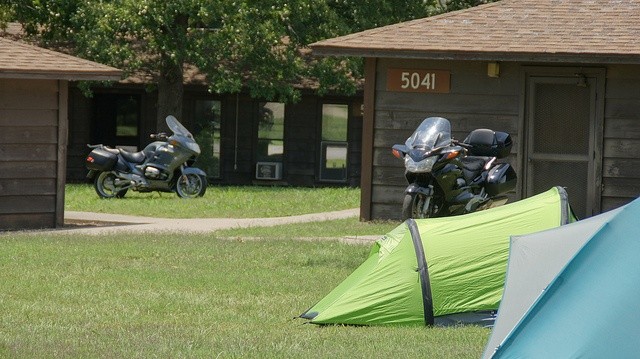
[[83, 115, 208, 199], [391, 117, 517, 218]]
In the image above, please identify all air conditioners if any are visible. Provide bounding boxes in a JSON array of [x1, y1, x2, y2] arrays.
[[255, 162, 282, 180]]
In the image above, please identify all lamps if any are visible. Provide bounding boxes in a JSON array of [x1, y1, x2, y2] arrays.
[[488, 61, 499, 78]]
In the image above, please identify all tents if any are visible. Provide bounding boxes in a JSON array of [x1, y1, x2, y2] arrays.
[[481, 194, 639, 358], [289, 184, 580, 328]]
